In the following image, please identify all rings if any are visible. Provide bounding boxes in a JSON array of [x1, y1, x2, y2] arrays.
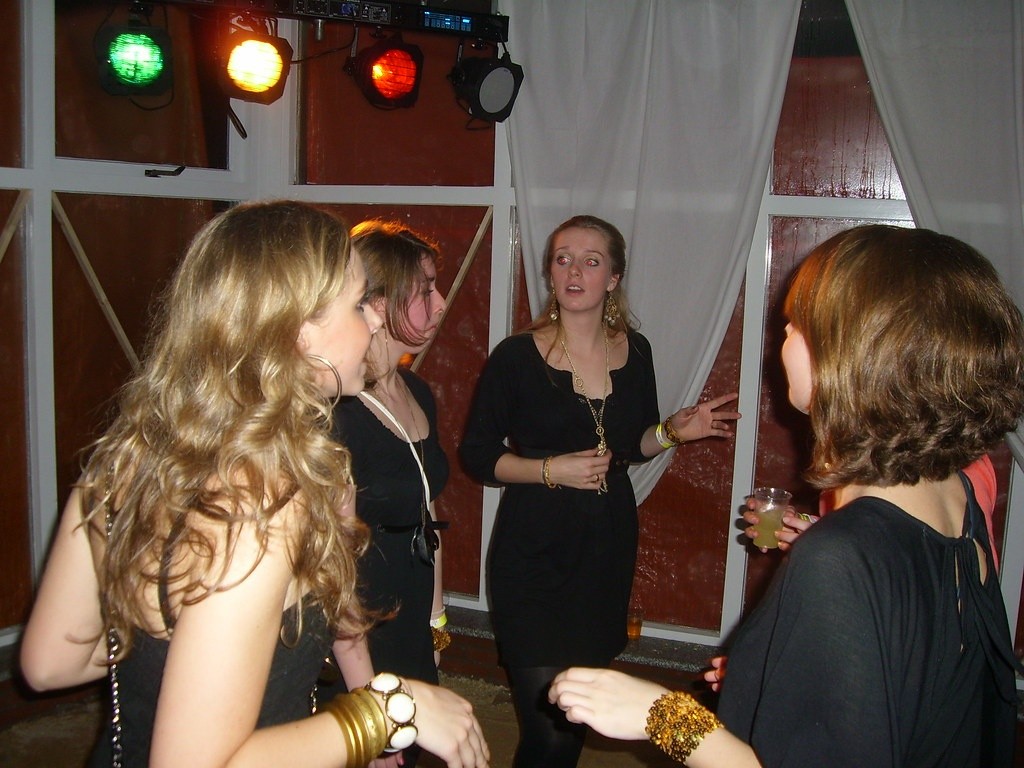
[[596, 474, 599, 481]]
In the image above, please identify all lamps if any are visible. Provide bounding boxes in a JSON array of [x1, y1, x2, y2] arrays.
[[343, 21, 423, 111], [445, 36, 523, 131], [95, 0, 175, 112], [207, 11, 294, 105]]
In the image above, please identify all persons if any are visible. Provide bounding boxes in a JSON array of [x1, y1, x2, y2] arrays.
[[20, 200, 490, 768], [458, 215, 742, 768], [548, 224, 1024, 768], [743, 454, 998, 571], [315, 220, 451, 768]]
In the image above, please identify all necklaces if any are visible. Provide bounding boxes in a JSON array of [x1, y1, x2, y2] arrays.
[[373, 376, 430, 554], [560, 323, 610, 494]]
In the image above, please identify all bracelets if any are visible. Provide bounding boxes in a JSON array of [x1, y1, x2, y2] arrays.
[[645, 690, 724, 763], [656, 424, 675, 448], [798, 512, 810, 521], [542, 456, 557, 488], [430, 605, 450, 651], [325, 672, 418, 768], [664, 415, 685, 445]]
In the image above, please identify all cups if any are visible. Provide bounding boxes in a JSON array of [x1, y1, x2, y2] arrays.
[[752, 487, 792, 549], [627, 609, 644, 640]]
